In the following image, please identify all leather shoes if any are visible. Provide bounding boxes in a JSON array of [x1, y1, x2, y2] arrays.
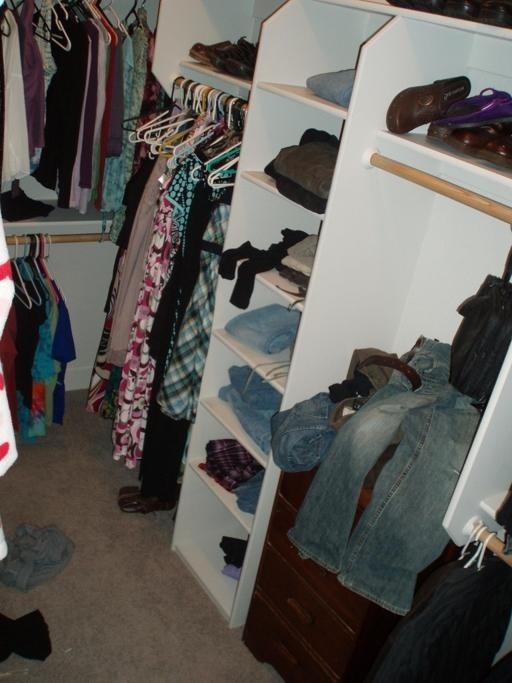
[[119, 487, 175, 514], [386, 77, 512, 167], [190, 36, 257, 78], [387, 0, 512, 26]]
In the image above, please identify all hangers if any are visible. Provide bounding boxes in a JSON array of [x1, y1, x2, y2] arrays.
[[0, 0, 147, 51], [122, 76, 247, 189], [458, 523, 497, 571], [10, 233, 59, 309]]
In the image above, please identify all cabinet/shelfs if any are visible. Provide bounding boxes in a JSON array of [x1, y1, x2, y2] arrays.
[[171, 0, 512, 683]]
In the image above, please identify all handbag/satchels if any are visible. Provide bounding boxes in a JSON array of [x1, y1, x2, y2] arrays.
[[449, 245, 511, 400]]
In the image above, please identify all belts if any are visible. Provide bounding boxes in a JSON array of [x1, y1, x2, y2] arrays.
[[356, 335, 427, 393]]
